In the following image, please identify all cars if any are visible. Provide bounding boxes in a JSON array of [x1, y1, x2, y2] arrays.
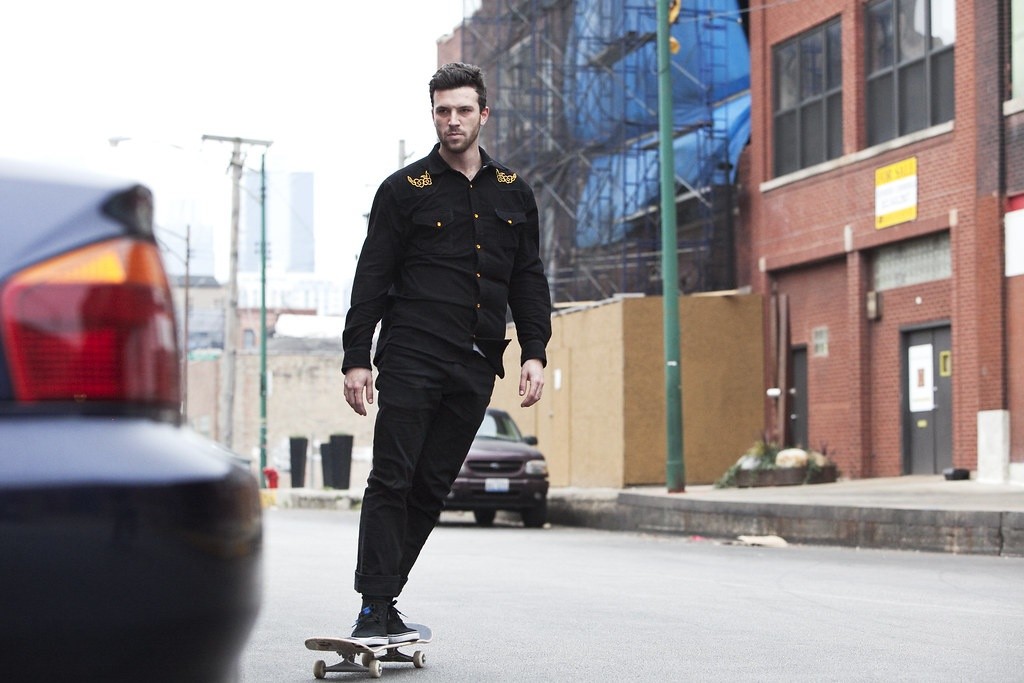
[[0, 168, 268, 683]]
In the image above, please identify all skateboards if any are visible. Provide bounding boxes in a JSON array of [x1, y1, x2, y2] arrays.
[[304, 622, 434, 679]]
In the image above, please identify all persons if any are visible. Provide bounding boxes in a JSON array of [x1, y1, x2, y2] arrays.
[[339, 64, 552, 647]]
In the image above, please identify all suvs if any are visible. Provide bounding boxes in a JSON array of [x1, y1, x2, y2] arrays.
[[440, 408, 550, 526]]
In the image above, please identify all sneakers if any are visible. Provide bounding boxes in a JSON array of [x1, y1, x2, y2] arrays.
[[350, 599, 420, 645]]
